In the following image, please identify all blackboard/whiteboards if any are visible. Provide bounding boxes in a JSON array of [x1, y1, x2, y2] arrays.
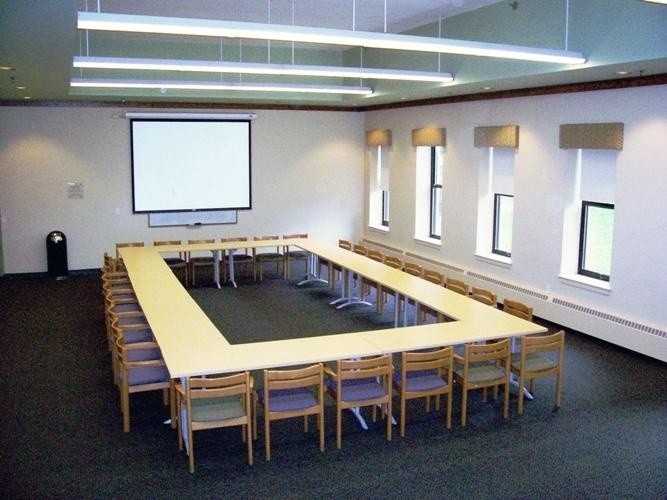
[[148, 210, 237, 227]]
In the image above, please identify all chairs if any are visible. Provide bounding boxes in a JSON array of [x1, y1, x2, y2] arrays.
[[115, 234, 311, 290], [504, 331, 566, 415], [453, 337, 513, 427], [394, 346, 452, 437], [176, 372, 254, 473], [318, 238, 533, 331], [102, 253, 170, 434], [257, 364, 326, 459], [323, 354, 393, 450]]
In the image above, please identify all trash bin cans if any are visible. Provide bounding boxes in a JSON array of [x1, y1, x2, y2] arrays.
[[46, 231, 69, 280]]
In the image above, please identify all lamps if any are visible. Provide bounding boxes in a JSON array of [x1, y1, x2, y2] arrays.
[[75, 0, 586, 67], [70, 68, 373, 98], [73, 13, 453, 87]]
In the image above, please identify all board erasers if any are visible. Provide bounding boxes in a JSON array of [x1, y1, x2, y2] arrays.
[[195, 223, 201, 225]]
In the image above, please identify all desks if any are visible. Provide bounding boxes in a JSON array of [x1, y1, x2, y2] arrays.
[[117, 240, 550, 430]]
